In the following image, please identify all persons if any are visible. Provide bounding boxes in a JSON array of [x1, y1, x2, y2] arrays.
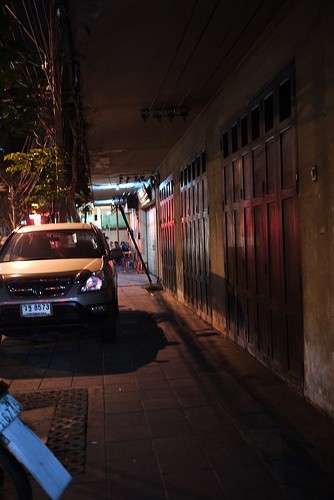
[[105, 236, 133, 266]]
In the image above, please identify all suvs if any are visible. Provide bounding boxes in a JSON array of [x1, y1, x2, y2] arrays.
[[1, 221, 118, 338]]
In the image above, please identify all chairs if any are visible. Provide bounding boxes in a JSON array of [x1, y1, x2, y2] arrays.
[[70, 239, 94, 259], [25, 238, 51, 259]]
